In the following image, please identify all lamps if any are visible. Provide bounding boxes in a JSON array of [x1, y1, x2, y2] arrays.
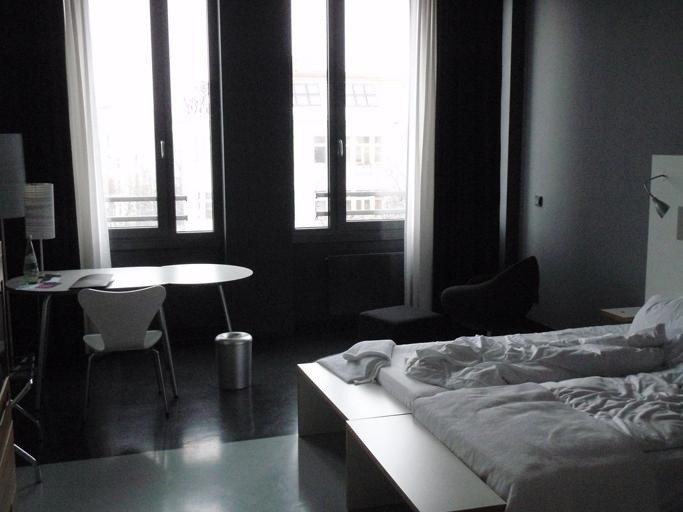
[[643, 174, 671, 219], [0, 133, 57, 280]]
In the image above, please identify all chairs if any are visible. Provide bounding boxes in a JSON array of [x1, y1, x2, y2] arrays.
[[77, 282, 180, 424]]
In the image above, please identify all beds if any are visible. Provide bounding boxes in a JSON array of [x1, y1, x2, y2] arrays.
[[364, 326, 683, 512]]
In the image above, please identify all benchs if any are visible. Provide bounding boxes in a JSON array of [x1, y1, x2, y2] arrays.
[[291, 359, 507, 511]]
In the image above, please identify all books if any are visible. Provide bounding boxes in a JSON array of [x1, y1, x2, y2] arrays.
[[69, 274, 114, 289]]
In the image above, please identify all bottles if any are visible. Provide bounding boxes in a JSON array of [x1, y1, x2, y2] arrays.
[[22, 233, 39, 284]]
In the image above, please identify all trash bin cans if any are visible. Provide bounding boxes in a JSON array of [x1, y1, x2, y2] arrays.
[[217, 332, 253, 390]]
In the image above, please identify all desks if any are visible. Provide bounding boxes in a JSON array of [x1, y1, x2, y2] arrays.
[[3, 262, 256, 414]]
